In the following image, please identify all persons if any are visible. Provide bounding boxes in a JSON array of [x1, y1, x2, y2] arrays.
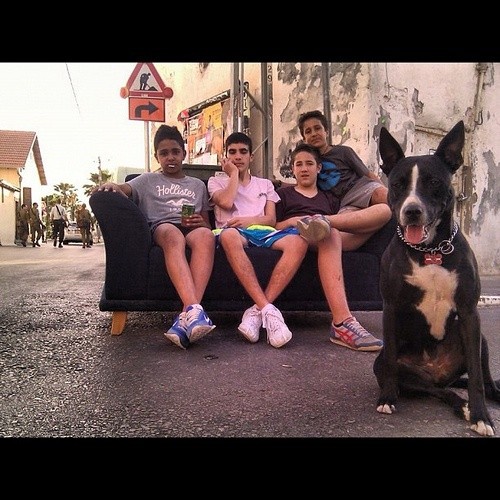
[[16, 204, 30, 247], [273, 143, 394, 352], [206, 133, 308, 349], [90, 126, 218, 350], [297, 110, 391, 215], [76, 204, 95, 249], [29, 204, 48, 248], [50, 199, 71, 248]]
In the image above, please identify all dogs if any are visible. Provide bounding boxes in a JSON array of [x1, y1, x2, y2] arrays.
[[373, 119, 499, 436]]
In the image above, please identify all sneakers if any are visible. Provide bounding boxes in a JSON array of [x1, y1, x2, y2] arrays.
[[297, 214, 331, 243], [164, 303, 216, 350], [329, 316, 383, 351], [238, 303, 293, 348]]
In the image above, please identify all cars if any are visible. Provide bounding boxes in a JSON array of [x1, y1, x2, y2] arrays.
[[63, 221, 93, 245]]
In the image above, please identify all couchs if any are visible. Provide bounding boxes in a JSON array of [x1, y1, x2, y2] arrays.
[[89, 174, 385, 335]]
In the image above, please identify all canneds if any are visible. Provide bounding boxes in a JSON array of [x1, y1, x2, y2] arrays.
[[181, 204, 196, 229]]
